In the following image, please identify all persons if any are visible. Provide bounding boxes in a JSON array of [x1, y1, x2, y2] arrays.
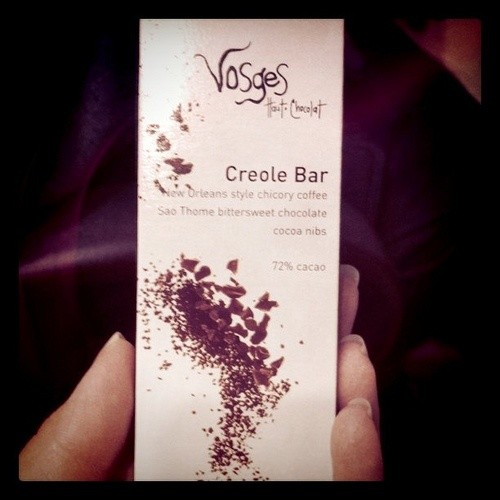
[[19, 264, 385, 484]]
